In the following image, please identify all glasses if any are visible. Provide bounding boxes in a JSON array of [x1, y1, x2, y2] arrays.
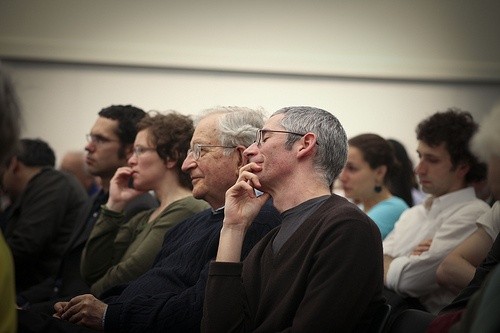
[[254, 128, 319, 147], [124, 145, 158, 158], [187, 143, 237, 161], [86, 134, 119, 144]]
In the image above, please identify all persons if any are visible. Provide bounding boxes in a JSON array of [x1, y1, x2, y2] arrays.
[[53, 106, 269, 332], [382, 108, 491, 333], [0, 67, 500, 333], [200, 105, 386, 332]]
